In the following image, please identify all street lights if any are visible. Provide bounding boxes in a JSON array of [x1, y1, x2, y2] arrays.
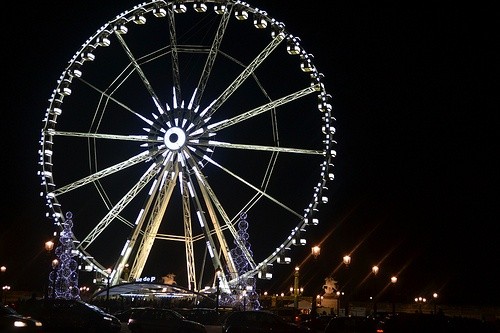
[[433, 293, 438, 311], [44, 241, 55, 300], [215, 265, 222, 321], [51, 258, 58, 298], [372, 265, 379, 316], [343, 255, 351, 318], [311, 244, 321, 314], [390, 276, 398, 317], [105, 267, 113, 311]]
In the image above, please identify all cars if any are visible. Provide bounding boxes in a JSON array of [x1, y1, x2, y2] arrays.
[[128, 308, 208, 333], [294, 313, 389, 333], [0, 299, 121, 333], [221, 309, 313, 333]]
